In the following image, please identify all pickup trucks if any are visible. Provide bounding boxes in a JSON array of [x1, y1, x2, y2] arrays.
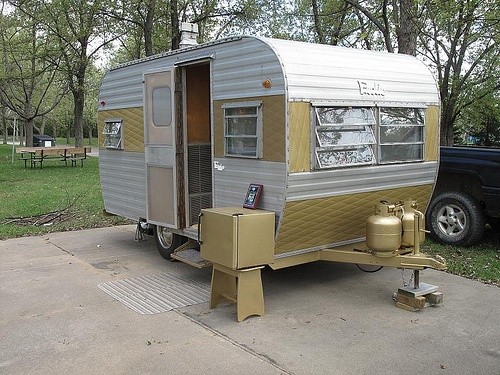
[[426, 145, 500, 246]]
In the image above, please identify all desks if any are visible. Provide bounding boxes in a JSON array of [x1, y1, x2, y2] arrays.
[[22, 147, 91, 168]]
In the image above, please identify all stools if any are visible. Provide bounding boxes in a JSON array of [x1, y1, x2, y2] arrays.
[[209, 262, 265, 322]]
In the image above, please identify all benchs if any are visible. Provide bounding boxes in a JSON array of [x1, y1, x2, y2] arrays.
[[16, 147, 84, 168], [33, 147, 75, 169]]
